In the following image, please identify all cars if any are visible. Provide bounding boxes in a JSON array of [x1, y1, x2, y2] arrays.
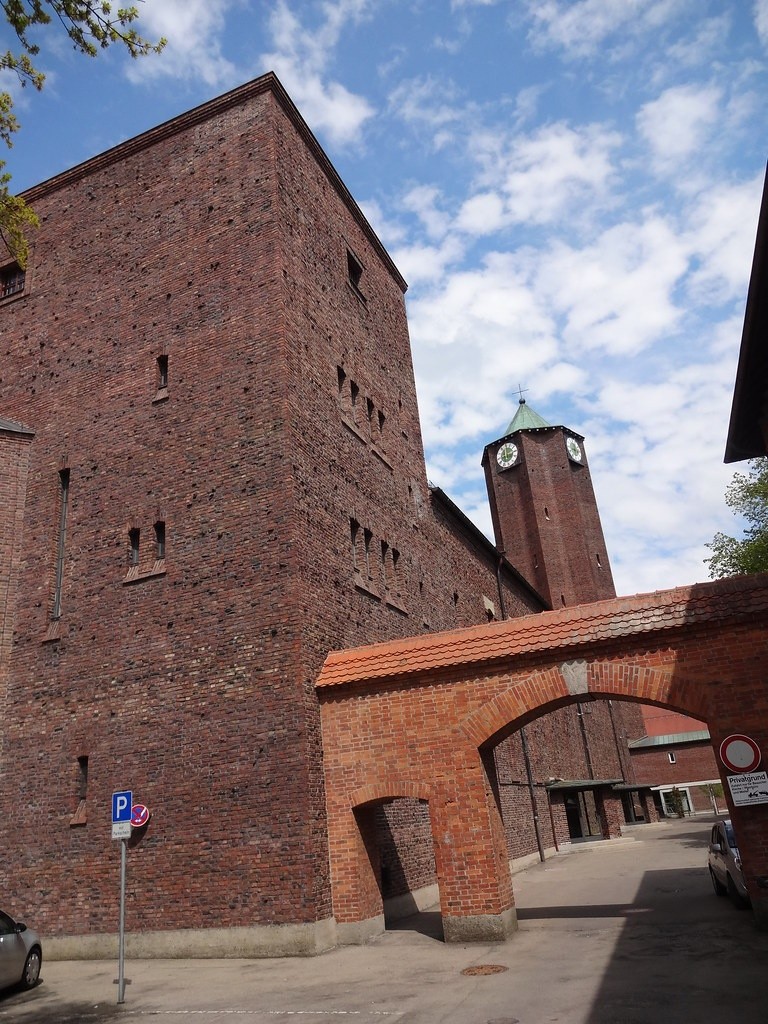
[[0, 910, 42, 989], [709, 819, 749, 910]]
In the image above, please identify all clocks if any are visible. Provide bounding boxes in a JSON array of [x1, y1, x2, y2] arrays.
[[567, 437, 582, 462], [496, 442, 518, 467]]
